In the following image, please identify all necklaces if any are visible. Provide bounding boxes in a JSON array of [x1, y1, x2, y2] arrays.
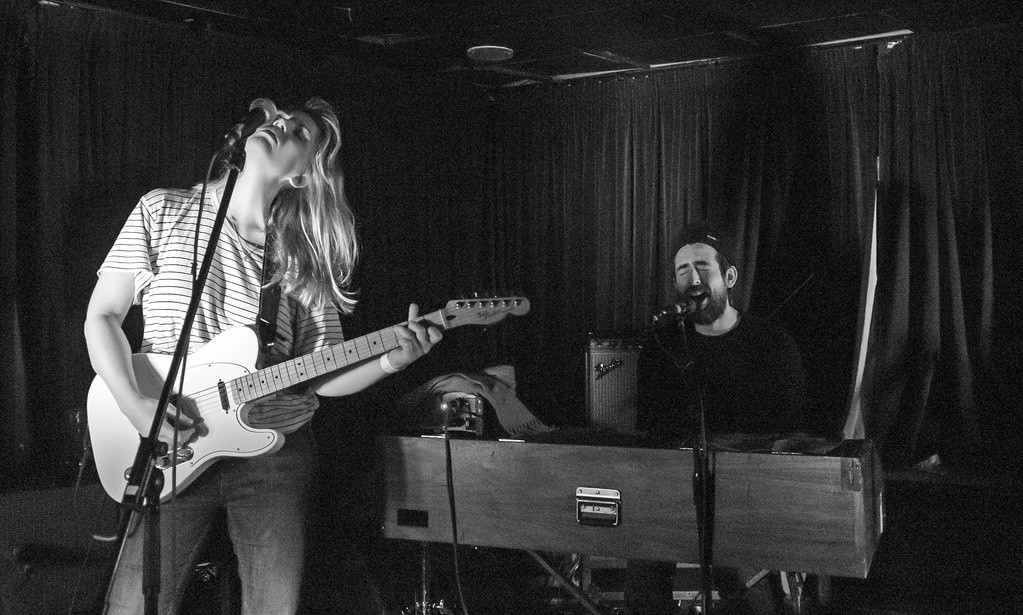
[[229, 209, 261, 260]]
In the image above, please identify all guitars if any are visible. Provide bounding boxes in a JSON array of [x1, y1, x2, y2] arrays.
[[84, 290, 534, 508]]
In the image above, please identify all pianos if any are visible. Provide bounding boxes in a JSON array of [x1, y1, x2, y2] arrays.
[[372, 422, 887, 581]]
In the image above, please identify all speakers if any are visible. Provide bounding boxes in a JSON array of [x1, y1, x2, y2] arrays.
[[585, 336, 652, 435]]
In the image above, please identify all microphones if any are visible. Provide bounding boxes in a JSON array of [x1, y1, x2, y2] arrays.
[[653, 301, 696, 321], [215, 98, 277, 154]]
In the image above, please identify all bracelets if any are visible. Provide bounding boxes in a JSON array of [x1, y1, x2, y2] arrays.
[[381, 350, 402, 374]]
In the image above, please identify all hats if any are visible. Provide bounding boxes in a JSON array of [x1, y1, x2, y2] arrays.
[[670, 234, 736, 266]]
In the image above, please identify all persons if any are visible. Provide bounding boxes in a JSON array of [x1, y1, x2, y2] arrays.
[[84, 98, 443, 615], [624, 224, 810, 615]]
